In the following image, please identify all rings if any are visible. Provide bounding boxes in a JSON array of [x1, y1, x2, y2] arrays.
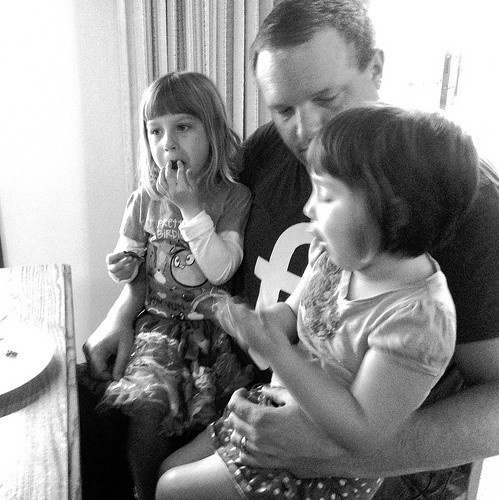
[[240, 435, 248, 451]]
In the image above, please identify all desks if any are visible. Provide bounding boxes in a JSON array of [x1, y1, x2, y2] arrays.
[[0, 262, 82, 500]]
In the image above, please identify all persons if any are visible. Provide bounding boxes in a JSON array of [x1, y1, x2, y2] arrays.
[[106, 71, 254, 500], [75, 0, 499, 500], [155, 100, 482, 500]]
